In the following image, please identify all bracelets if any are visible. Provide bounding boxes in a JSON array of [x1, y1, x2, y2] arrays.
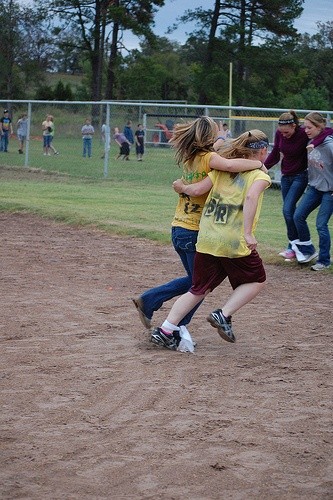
[[260, 162, 263, 169], [217, 136, 226, 140]]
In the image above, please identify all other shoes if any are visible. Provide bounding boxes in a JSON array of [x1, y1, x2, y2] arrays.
[[131, 295, 152, 329], [138, 158, 142, 161], [176, 337, 197, 347], [19, 149, 23, 154]]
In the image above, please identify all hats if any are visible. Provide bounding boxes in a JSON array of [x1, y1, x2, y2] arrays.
[[4, 110, 8, 113]]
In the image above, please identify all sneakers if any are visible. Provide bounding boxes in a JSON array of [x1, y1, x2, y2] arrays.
[[279, 249, 296, 261], [299, 250, 318, 263], [206, 308, 237, 343], [311, 263, 330, 271], [149, 327, 177, 350]]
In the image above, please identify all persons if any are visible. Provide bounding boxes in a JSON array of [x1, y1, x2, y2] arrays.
[[135, 123, 144, 160], [151, 129, 268, 349], [123, 120, 135, 160], [221, 124, 232, 137], [99, 124, 110, 158], [132, 115, 268, 344], [41, 115, 56, 156], [292, 112, 333, 271], [263, 111, 333, 258], [114, 127, 130, 160], [16, 114, 28, 154], [0, 109, 13, 152], [80, 120, 95, 158]]
